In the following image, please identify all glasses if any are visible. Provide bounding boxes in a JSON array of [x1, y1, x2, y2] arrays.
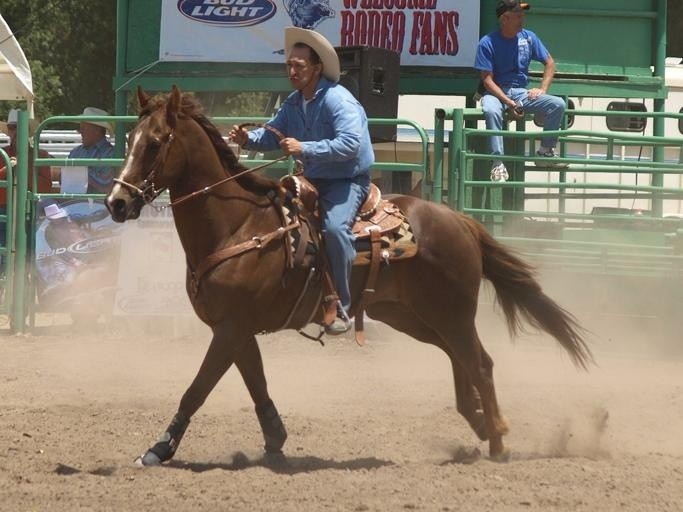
[[7, 124, 17, 131]]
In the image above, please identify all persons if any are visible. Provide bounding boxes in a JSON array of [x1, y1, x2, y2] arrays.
[[227, 26, 376, 334], [0, 108, 52, 267], [473, 0, 569, 185], [32, 107, 117, 311]]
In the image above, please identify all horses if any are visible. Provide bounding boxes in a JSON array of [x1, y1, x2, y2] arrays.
[[100, 81, 595, 466]]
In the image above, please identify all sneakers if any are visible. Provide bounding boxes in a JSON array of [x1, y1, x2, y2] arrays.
[[490, 162, 509, 182], [325, 316, 352, 333], [535, 152, 568, 168]]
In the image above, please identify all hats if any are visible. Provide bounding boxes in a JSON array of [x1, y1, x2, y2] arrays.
[[496, 0, 530, 17], [70, 107, 115, 130], [38, 205, 69, 219], [0, 109, 41, 138], [284, 27, 341, 83]]
